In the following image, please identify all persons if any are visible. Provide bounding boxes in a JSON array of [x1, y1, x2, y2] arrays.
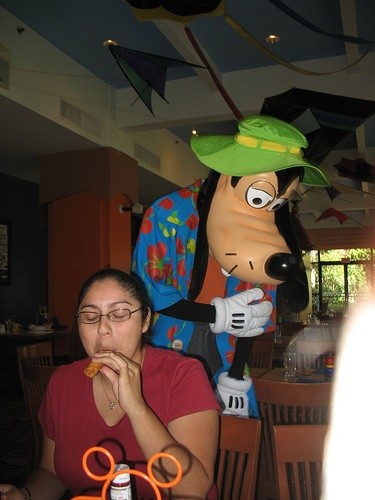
[[0, 268, 222, 500]]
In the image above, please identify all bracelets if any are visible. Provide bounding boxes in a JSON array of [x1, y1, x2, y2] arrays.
[[20, 487, 32, 500]]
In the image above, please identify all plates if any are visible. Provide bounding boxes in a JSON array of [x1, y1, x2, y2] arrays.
[[27, 331, 53, 336]]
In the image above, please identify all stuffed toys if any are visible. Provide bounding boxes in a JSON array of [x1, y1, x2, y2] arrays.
[[131, 116, 330, 500]]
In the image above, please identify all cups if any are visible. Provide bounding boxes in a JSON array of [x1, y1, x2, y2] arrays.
[[282, 352, 298, 377], [5, 319, 16, 335]]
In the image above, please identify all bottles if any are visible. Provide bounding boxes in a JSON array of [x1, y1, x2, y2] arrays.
[[110, 463, 132, 500]]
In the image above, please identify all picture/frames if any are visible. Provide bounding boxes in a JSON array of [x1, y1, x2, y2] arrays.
[[0, 220, 13, 286]]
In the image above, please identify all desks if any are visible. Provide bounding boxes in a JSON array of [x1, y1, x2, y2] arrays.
[[262, 367, 325, 383], [0, 328, 73, 355]]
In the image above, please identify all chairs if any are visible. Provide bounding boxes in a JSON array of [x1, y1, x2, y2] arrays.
[[214, 336, 332, 500], [16, 324, 89, 471]]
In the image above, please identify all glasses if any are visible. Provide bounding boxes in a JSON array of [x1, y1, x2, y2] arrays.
[[74, 307, 142, 324]]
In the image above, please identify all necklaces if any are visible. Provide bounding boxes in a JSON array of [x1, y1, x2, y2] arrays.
[[98, 373, 118, 410]]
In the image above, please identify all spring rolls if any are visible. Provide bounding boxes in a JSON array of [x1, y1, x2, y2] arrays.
[[83, 361, 103, 378]]
